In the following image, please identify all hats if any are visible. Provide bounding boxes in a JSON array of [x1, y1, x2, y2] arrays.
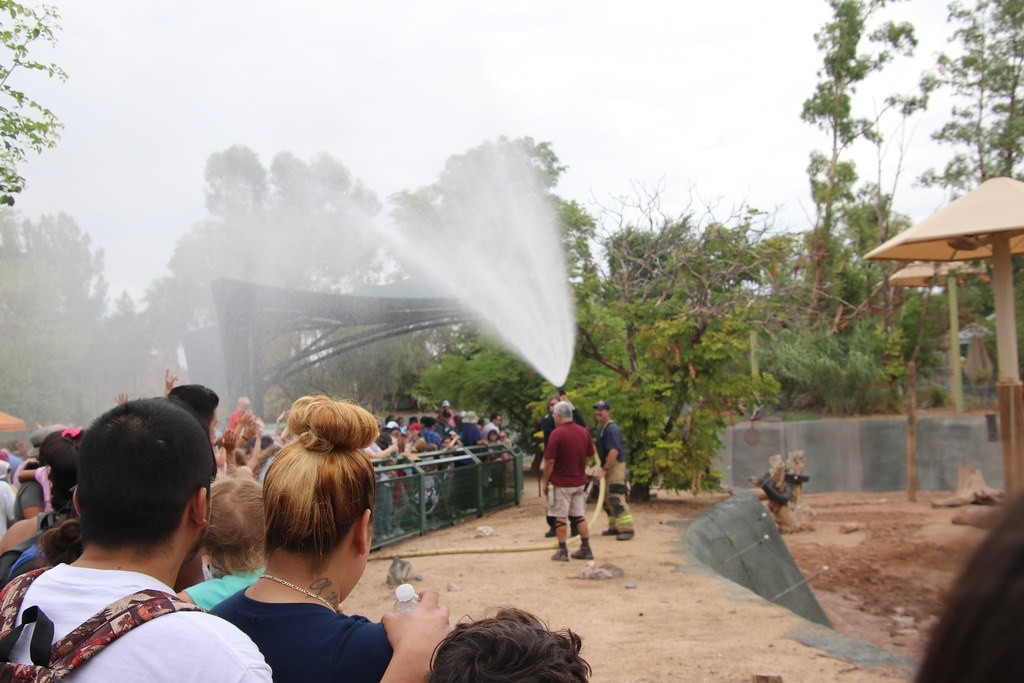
[[442, 400, 450, 408], [27, 424, 66, 459], [408, 422, 424, 432], [381, 420, 401, 431], [592, 400, 611, 411], [461, 411, 480, 425]]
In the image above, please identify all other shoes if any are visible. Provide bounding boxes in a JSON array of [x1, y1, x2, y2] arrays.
[[571, 546, 594, 560], [570, 523, 578, 536], [617, 532, 635, 541], [546, 529, 556, 536], [551, 549, 571, 562], [601, 530, 618, 536]]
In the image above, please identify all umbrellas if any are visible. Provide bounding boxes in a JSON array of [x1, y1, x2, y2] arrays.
[[864, 177, 1024, 381], [888, 260, 989, 410]]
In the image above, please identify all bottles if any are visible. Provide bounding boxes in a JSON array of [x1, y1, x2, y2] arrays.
[[392, 583, 422, 618]]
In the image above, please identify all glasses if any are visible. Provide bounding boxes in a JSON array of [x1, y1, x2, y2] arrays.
[[550, 403, 556, 406]]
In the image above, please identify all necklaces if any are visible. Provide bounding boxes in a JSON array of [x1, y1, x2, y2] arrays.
[[260, 575, 336, 612]]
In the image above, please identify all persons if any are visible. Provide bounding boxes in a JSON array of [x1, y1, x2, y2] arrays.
[[428, 608, 592, 683], [0, 370, 292, 610], [914, 491, 1024, 683], [541, 395, 595, 561], [592, 401, 635, 540], [364, 402, 512, 551], [0, 399, 452, 683], [207, 397, 394, 683]]
